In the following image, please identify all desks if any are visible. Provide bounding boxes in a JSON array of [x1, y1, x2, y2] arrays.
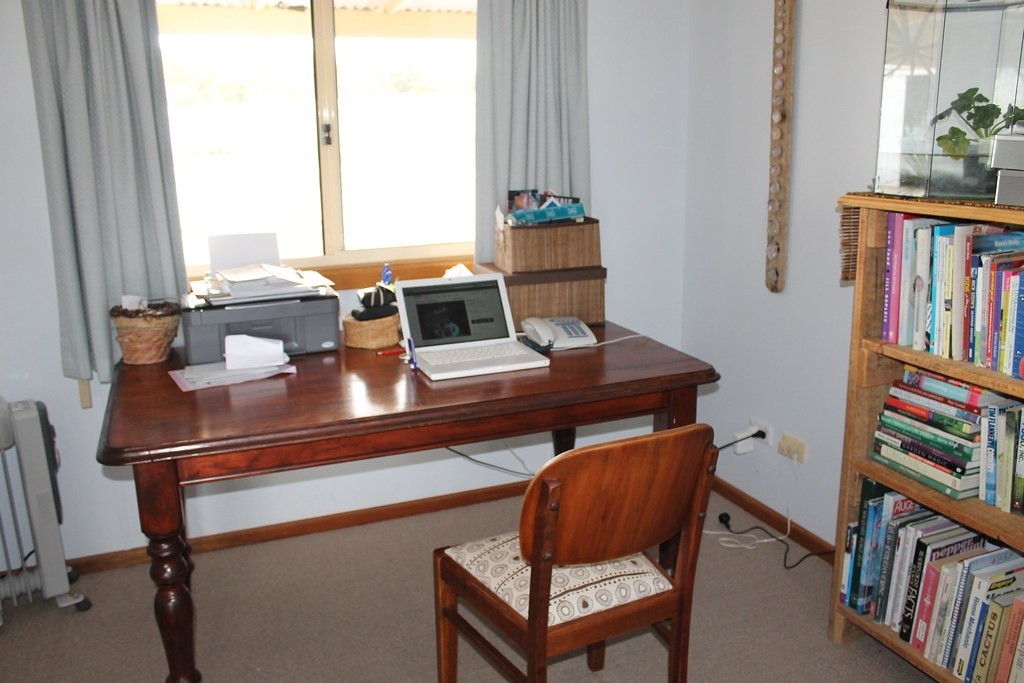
[[96, 319, 721, 683]]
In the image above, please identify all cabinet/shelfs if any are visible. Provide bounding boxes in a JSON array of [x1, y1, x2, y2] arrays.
[[829, 192, 1024, 683]]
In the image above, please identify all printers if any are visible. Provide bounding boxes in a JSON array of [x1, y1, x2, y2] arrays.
[[183, 285, 341, 366]]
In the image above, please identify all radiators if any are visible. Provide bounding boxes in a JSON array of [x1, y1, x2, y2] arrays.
[[0, 396, 93, 629]]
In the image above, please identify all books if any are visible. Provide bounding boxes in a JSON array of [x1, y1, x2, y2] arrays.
[[839, 477, 1024, 683], [871, 364, 1024, 515], [883, 213, 1024, 380]]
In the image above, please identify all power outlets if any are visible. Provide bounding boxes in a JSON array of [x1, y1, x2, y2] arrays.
[[755, 423, 773, 444]]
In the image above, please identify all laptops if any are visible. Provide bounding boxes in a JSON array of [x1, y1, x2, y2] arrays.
[[395, 272, 551, 380]]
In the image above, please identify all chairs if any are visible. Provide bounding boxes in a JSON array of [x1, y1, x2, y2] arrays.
[[433, 423, 721, 683]]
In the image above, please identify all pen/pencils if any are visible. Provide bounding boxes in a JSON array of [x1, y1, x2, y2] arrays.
[[376, 349, 406, 356]]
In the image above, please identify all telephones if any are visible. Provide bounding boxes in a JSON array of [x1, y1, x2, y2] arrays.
[[521, 316, 597, 351]]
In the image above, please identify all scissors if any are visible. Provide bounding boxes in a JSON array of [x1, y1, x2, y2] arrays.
[[382, 262, 392, 284]]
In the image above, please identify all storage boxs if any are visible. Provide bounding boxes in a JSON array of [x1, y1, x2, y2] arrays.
[[477, 261, 607, 329], [183, 296, 341, 364], [492, 215, 601, 272]]
